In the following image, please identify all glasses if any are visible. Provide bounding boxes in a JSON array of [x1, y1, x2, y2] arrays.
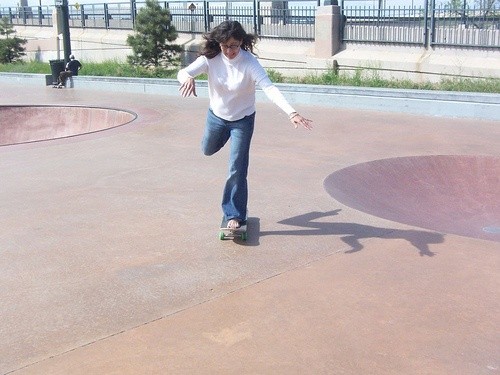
[[220, 41, 240, 49]]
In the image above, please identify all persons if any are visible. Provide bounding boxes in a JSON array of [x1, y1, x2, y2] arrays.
[[176, 20, 313, 229], [53, 55, 83, 87]]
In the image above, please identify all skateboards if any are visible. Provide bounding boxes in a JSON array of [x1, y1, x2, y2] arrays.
[[220, 215, 247, 241], [52, 85, 64, 89]]
